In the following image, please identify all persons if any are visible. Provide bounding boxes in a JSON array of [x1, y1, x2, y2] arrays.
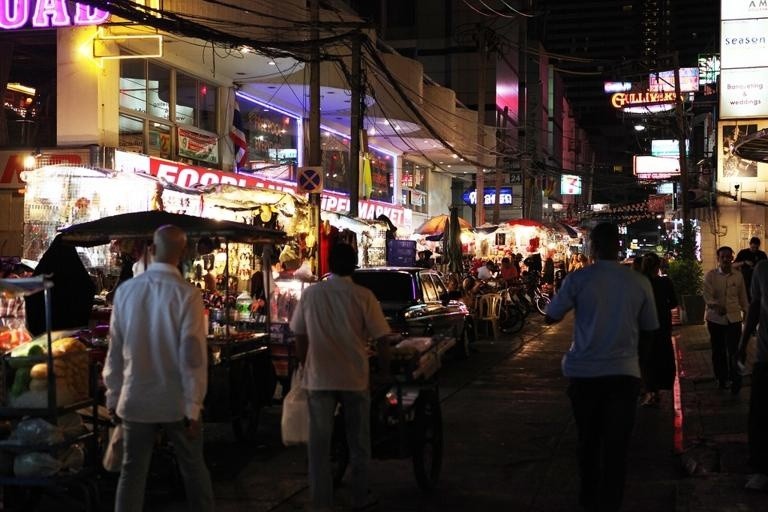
[[630, 252, 678, 406], [288, 244, 391, 512], [702, 236, 768, 400], [544, 220, 664, 512], [101, 225, 218, 512], [735, 259, 767, 488], [417, 246, 590, 313]]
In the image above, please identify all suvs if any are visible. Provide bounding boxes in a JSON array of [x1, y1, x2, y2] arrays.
[[350, 267, 474, 387]]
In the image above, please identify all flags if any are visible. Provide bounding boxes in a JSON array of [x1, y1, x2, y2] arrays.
[[226, 87, 249, 172]]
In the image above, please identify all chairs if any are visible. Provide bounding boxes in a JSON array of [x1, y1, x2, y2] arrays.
[[471, 291, 504, 344]]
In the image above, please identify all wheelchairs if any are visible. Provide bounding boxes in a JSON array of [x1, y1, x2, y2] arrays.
[[302, 340, 445, 494]]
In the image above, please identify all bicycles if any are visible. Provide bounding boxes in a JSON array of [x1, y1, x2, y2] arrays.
[[446, 269, 554, 335]]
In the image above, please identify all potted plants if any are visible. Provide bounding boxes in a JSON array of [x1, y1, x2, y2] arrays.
[[664, 258, 707, 326]]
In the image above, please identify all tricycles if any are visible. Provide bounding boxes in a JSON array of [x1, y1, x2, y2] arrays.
[[57, 213, 288, 452]]
